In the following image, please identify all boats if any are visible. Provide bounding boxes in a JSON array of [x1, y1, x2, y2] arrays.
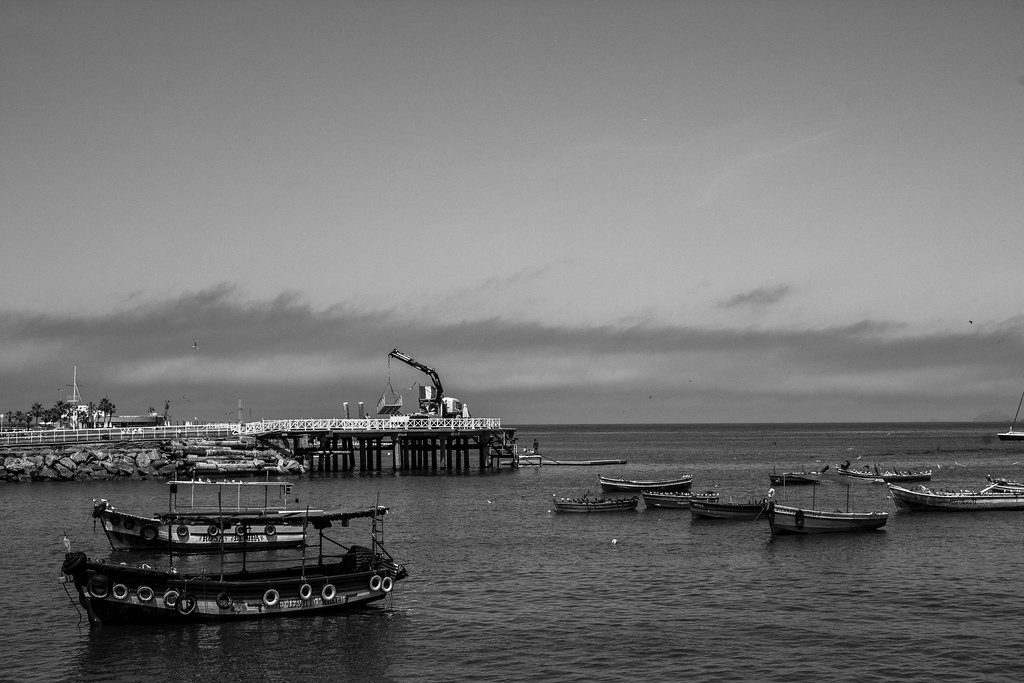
[[888, 482, 1024, 512], [882, 466, 933, 484], [763, 474, 890, 533], [597, 473, 694, 492], [90, 461, 308, 552], [767, 464, 830, 483], [688, 489, 778, 519], [640, 490, 720, 510], [985, 474, 1024, 494], [57, 491, 410, 625], [836, 459, 885, 479], [551, 494, 639, 513]]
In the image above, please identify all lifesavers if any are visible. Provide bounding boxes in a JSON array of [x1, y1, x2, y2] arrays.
[[88, 577, 110, 599], [163, 589, 179, 608], [138, 585, 154, 604], [321, 584, 337, 601], [369, 575, 382, 592], [300, 584, 312, 601], [262, 589, 280, 606], [216, 592, 233, 609], [177, 525, 188, 537], [113, 584, 128, 600], [110, 515, 121, 526], [176, 594, 197, 616], [382, 576, 393, 593], [124, 519, 135, 530], [265, 524, 276, 536], [92, 502, 106, 518], [208, 526, 220, 536], [140, 523, 159, 542], [235, 525, 246, 536]]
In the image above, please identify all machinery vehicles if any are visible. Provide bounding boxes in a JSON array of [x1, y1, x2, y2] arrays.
[[374, 349, 471, 422]]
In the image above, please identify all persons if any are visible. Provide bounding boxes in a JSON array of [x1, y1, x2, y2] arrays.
[[365, 413, 373, 428], [533, 439, 538, 454]]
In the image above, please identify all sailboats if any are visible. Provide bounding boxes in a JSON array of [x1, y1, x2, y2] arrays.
[[997, 393, 1024, 440]]
